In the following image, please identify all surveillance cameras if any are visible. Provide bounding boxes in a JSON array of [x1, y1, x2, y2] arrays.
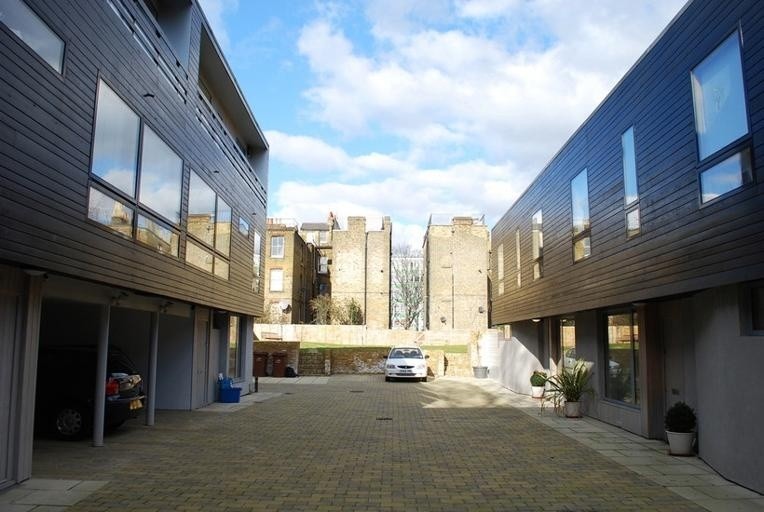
[[478, 306, 486, 313]]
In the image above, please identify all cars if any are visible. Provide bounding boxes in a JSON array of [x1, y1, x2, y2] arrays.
[[383, 346, 430, 382], [564, 346, 622, 379], [33, 326, 147, 442]]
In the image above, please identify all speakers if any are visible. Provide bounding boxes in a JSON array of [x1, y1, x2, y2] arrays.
[[212, 313, 228, 330]]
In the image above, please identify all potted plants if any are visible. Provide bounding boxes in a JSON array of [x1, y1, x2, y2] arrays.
[[527, 360, 599, 419], [662, 401, 697, 456]]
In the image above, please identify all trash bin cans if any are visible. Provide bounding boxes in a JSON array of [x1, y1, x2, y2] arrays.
[[254, 352, 267, 376], [473, 366, 487, 379], [273, 352, 286, 377]]
